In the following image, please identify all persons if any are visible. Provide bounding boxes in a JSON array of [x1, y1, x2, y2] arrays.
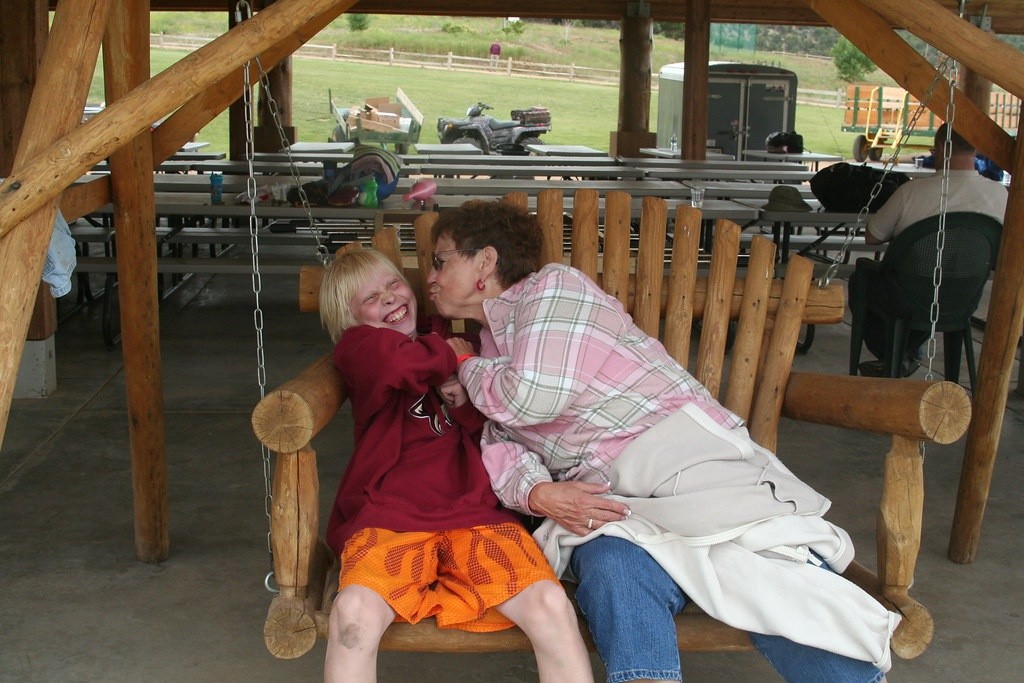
[[488, 38, 502, 69], [427, 198, 902, 683], [847, 122, 1008, 378], [320, 247, 594, 683]]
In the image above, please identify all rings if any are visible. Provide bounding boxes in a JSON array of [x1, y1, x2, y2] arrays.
[[588, 519, 594, 529]]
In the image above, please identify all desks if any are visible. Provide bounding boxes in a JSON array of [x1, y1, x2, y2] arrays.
[[41, 105, 938, 356]]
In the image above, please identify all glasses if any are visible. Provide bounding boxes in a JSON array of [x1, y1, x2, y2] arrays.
[[431, 249, 479, 272], [929, 147, 938, 155]]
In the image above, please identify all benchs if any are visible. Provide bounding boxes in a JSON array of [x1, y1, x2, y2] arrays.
[[154, 225, 366, 249], [59, 256, 124, 353], [155, 256, 340, 276], [74, 200, 115, 223], [636, 261, 858, 353], [69, 226, 116, 255], [737, 232, 895, 261]]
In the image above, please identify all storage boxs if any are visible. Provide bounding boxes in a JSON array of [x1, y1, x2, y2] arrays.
[[346, 97, 403, 134]]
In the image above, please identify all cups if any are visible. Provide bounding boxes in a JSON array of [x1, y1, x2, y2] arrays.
[[210, 175, 224, 203], [1002, 170, 1011, 186], [915, 158, 924, 169], [690, 188, 706, 207]]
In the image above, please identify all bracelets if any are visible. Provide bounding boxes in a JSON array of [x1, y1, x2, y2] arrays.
[[456, 352, 480, 364]]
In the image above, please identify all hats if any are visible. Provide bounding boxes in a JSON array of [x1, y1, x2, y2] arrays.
[[760, 185, 813, 212]]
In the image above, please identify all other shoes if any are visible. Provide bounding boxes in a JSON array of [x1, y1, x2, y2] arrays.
[[857, 355, 924, 378]]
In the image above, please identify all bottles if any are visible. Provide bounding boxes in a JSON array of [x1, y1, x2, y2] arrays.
[[670, 133, 678, 152], [402, 177, 437, 202], [364, 180, 379, 209]]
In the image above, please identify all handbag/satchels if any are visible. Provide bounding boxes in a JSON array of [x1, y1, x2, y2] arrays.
[[325, 145, 405, 204]]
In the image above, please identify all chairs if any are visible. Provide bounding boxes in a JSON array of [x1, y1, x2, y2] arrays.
[[848, 210, 1003, 404]]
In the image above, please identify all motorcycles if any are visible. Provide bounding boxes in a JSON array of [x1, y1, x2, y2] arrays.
[[437, 102, 552, 157]]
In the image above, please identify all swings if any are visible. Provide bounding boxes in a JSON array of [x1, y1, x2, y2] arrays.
[[231, 0, 973, 663]]
